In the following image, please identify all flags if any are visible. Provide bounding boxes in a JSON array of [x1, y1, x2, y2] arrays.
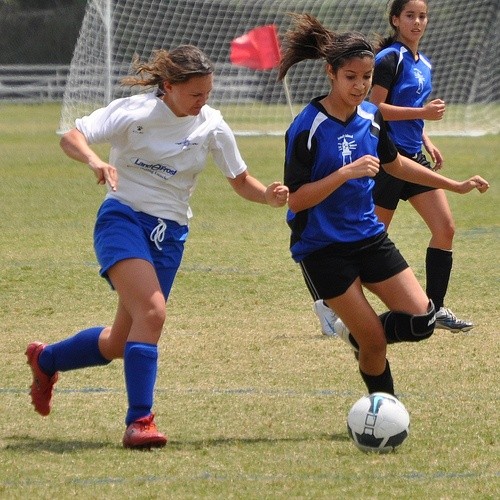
[[231, 26, 282, 71]]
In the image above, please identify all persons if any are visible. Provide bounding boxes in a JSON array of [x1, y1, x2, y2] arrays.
[[278, 12, 490, 396], [25, 46, 289, 450], [304, 0, 474, 336]]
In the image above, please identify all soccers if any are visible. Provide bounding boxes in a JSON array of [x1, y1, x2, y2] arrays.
[[346, 391, 410, 455]]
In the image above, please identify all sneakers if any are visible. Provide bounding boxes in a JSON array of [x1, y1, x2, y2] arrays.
[[314, 299, 339, 336], [24, 342, 58, 416], [122, 413, 167, 448], [435, 308, 474, 333], [334, 318, 360, 362]]
